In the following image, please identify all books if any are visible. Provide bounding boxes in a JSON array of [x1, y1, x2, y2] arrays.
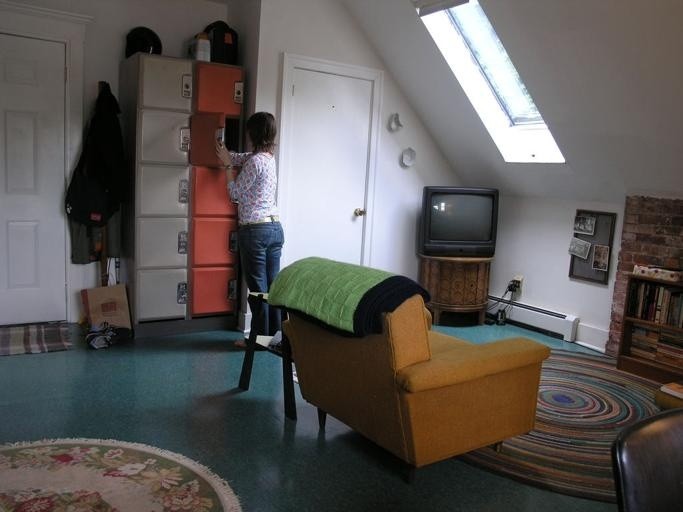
[[660, 381, 683, 401], [624, 279, 683, 369]]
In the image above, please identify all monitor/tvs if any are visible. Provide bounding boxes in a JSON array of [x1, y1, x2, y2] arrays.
[[418, 186, 498, 257]]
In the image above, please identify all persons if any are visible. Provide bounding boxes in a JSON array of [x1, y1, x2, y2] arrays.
[[214, 111, 284, 349]]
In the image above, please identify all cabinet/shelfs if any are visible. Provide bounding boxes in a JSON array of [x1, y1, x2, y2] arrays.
[[109, 50, 249, 343], [608, 262, 683, 388], [413, 249, 494, 329]]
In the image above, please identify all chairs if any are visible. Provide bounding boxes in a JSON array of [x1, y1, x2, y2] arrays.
[[605, 405, 682, 511]]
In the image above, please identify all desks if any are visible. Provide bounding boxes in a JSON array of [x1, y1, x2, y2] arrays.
[[235, 288, 301, 424]]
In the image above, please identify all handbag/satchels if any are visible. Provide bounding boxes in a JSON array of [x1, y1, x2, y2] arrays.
[[204, 21, 238, 65], [81, 283, 133, 338]]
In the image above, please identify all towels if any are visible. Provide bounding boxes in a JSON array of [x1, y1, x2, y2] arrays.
[[265, 255, 432, 340]]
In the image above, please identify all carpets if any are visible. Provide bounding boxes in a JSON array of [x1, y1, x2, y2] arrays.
[[0, 430, 242, 512], [452, 339, 674, 509], [0, 318, 73, 361]]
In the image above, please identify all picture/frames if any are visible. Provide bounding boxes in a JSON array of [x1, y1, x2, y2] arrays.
[[564, 206, 618, 289]]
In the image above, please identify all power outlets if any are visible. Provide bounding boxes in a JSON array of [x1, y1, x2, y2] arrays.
[[507, 275, 525, 302]]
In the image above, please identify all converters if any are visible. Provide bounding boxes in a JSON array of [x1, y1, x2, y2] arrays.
[[511, 284, 516, 292]]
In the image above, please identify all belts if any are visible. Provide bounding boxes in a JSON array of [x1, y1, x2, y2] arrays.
[[238, 216, 279, 226]]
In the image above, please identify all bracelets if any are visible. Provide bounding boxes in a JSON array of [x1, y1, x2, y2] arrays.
[[226, 165, 232, 168]]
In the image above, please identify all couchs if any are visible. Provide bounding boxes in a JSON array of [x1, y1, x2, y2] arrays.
[[262, 253, 554, 485]]
